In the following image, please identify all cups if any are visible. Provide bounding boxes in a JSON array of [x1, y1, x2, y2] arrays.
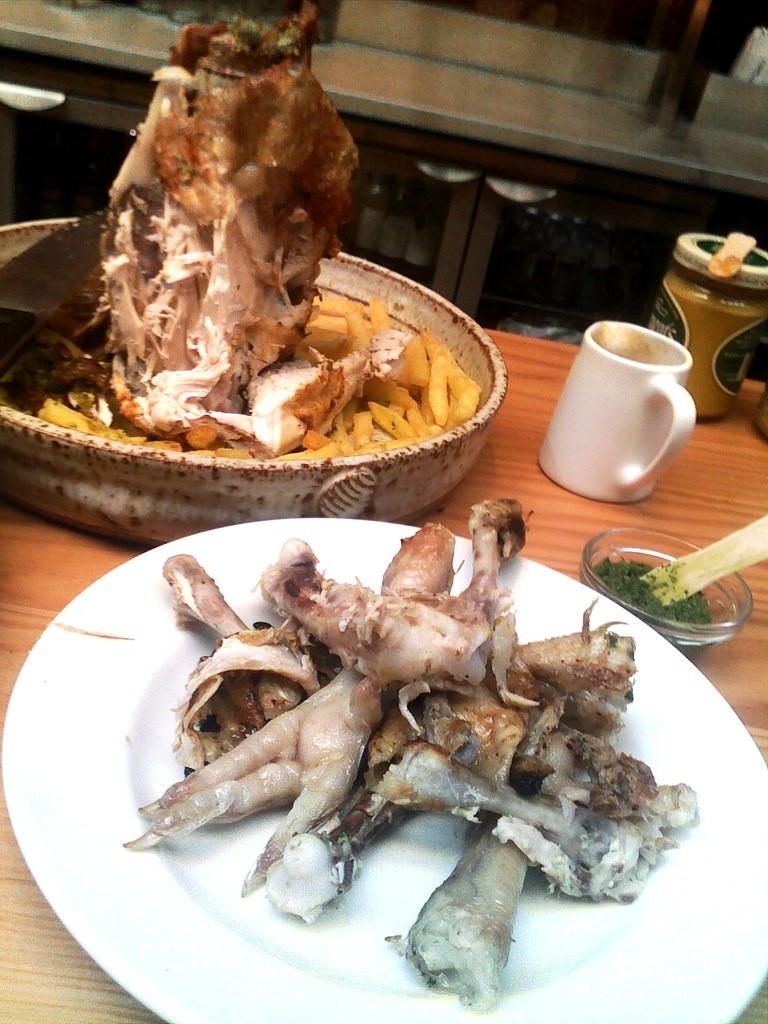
[[538, 323, 698, 503]]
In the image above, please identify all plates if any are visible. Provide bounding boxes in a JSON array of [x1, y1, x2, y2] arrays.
[[0, 516, 768, 1024]]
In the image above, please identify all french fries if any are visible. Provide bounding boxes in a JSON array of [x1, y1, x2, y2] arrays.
[[26, 292, 482, 453]]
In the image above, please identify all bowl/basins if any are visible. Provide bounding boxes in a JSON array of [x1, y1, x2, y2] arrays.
[[579, 526, 754, 658], [1, 216, 510, 549]]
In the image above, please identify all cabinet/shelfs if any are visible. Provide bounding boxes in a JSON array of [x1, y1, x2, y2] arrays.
[[0, 51, 768, 325]]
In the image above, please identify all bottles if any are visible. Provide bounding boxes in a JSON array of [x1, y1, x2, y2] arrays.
[[348, 172, 451, 284]]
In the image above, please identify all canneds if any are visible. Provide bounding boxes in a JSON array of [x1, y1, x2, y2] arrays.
[[647, 232, 768, 419]]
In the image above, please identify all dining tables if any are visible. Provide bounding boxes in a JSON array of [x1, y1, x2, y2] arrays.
[[0, 324, 768, 1024]]
